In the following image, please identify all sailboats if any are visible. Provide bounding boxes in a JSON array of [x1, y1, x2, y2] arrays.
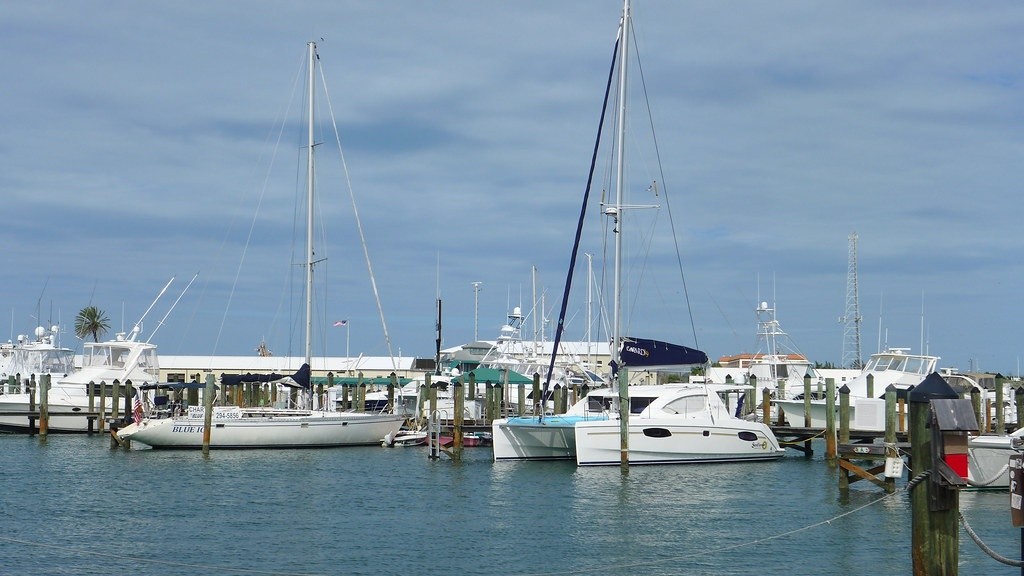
[[492, 0, 787, 467], [116, 41, 407, 446]]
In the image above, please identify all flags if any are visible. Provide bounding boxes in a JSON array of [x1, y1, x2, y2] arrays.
[[334, 321, 347, 325], [134, 396, 141, 425]]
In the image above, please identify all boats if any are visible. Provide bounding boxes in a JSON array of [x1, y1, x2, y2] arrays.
[[0, 270, 200, 431], [770, 290, 941, 431], [330, 249, 689, 432], [688, 271, 838, 422]]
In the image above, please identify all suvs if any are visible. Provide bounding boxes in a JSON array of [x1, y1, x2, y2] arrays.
[[940, 375, 1018, 433]]
